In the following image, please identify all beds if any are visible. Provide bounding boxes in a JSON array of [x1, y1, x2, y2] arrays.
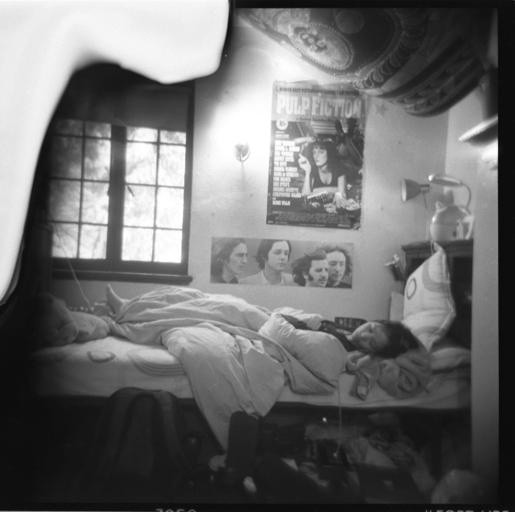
[[32, 339, 471, 482]]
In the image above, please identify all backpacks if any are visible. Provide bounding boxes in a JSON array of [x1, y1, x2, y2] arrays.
[[89, 387, 204, 499]]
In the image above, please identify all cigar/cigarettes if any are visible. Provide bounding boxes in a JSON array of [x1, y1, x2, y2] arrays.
[[299, 153, 303, 158]]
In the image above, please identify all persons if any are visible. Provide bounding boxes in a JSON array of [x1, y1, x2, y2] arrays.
[[291, 252, 328, 288], [320, 245, 351, 288], [104, 284, 419, 359], [240, 239, 296, 286], [297, 139, 346, 206], [211, 237, 248, 284]]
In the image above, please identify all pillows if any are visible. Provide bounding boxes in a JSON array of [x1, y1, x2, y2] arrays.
[[403, 244, 455, 352]]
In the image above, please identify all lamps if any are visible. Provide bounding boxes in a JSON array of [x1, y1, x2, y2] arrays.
[[401, 174, 472, 241]]
